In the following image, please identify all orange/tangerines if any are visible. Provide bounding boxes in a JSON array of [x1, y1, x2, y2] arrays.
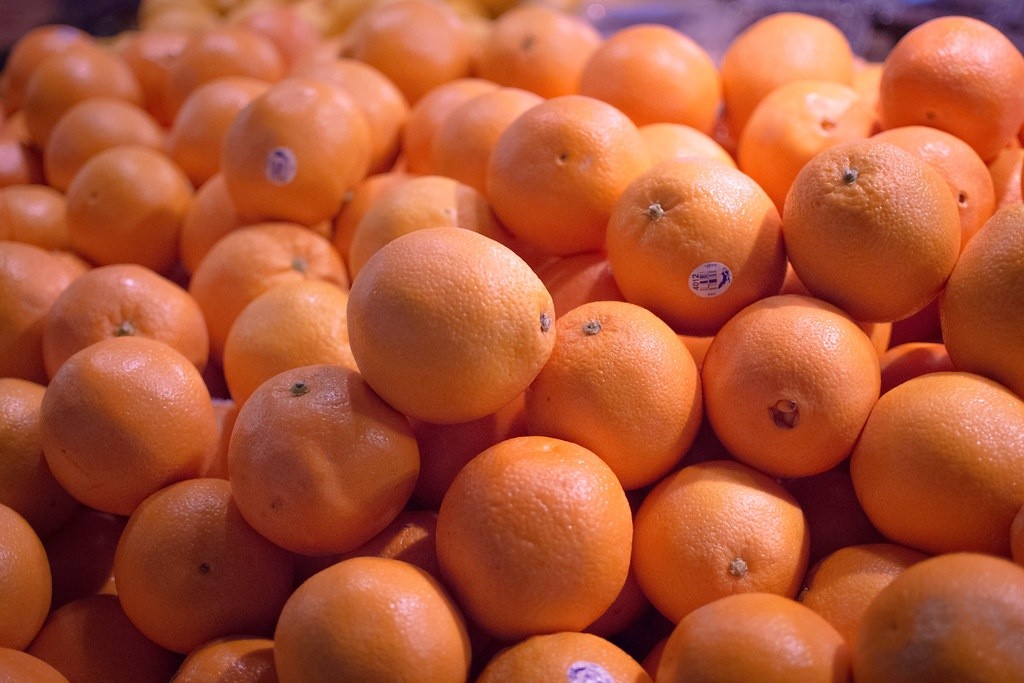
[[0, 0, 1024, 683]]
